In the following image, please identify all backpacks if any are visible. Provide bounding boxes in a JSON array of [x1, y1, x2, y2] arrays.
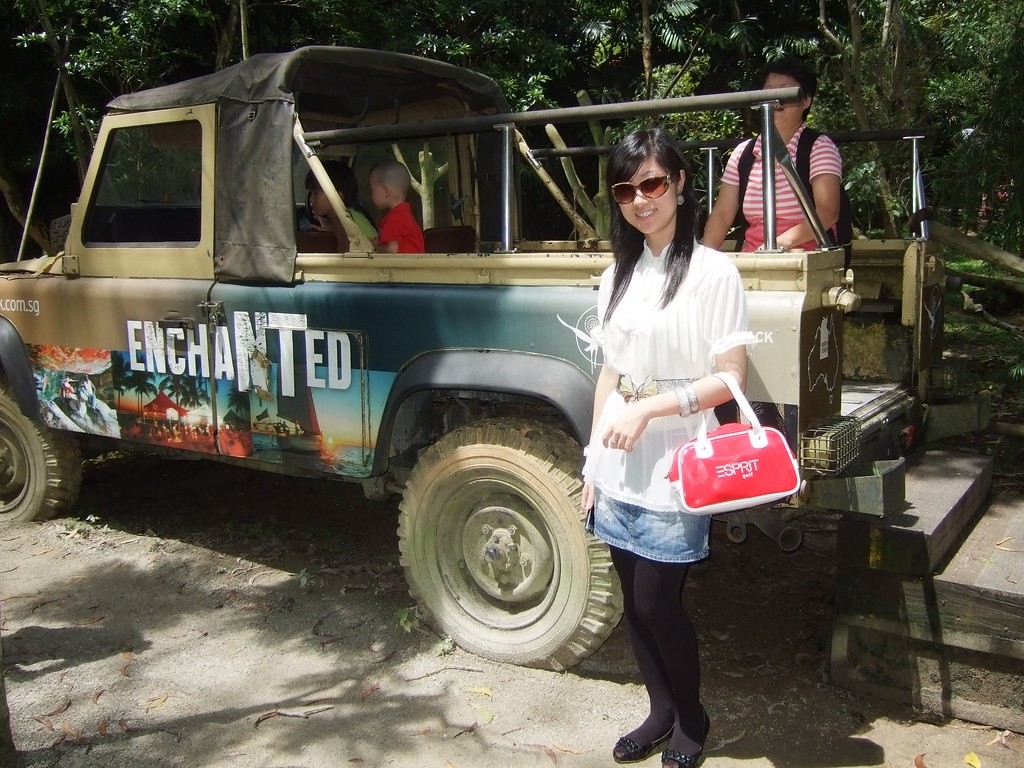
[[734, 127, 854, 276]]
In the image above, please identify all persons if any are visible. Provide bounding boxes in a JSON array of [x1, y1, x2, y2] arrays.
[[702, 56, 842, 253], [579, 127, 759, 768], [305, 160, 380, 253], [369, 161, 425, 254]]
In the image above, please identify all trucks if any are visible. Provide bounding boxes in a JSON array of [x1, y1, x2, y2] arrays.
[[0, 43, 995, 672]]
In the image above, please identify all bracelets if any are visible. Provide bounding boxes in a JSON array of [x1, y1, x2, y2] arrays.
[[684, 382, 699, 414], [675, 387, 691, 417]]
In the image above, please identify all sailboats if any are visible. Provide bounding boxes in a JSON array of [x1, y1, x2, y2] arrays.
[[272, 361, 325, 454]]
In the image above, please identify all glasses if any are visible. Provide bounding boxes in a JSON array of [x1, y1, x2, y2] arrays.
[[611, 173, 670, 204]]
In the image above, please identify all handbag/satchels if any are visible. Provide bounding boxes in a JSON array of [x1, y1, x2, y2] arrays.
[[664, 372, 801, 517]]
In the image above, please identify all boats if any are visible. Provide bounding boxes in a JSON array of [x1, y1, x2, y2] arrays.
[[249, 408, 293, 451]]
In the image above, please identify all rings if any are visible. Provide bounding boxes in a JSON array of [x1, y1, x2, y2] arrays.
[[611, 439, 617, 443]]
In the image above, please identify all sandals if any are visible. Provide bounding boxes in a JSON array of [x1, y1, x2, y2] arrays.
[[612, 722, 675, 764], [660, 703, 711, 768]]
